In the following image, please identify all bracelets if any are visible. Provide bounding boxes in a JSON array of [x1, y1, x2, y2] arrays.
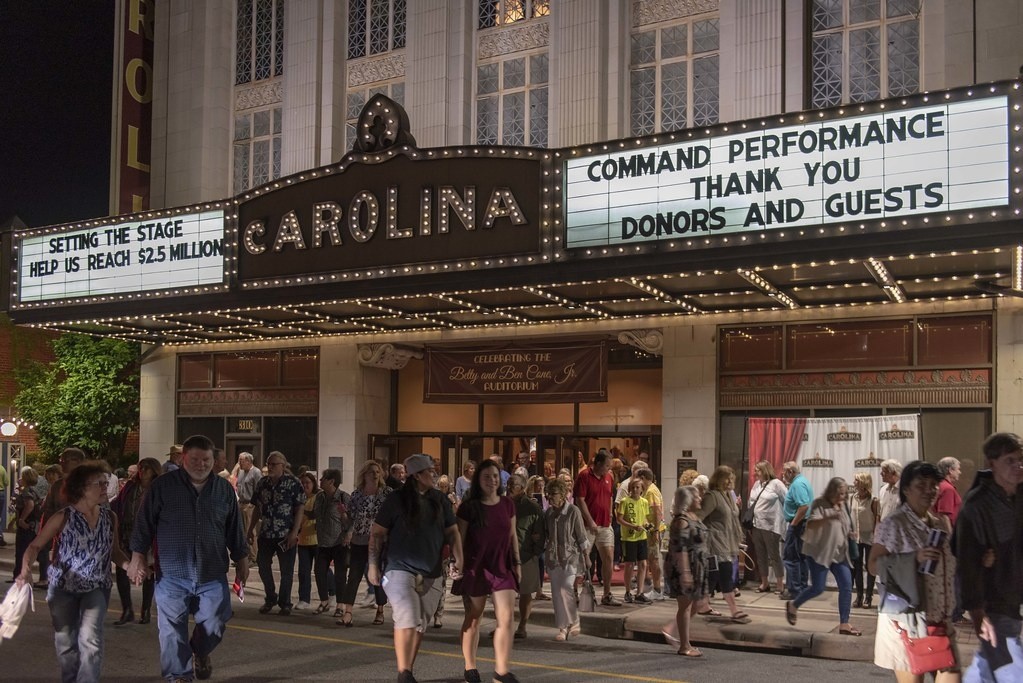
[[653, 530, 659, 532], [447, 559, 455, 568], [583, 553, 589, 557], [678, 568, 691, 575], [513, 561, 522, 566]]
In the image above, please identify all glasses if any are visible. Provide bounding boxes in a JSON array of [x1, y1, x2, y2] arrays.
[[266, 462, 283, 466], [91, 481, 109, 487]]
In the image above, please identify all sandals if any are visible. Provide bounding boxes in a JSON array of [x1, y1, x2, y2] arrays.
[[336, 611, 353, 627], [372, 612, 384, 625], [556, 616, 580, 641]]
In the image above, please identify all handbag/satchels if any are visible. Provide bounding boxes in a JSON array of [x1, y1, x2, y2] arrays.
[[741, 501, 756, 529], [899, 630, 955, 675], [580, 568, 597, 612]]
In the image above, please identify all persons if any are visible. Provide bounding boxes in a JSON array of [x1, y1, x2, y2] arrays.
[[16, 462, 146, 683], [677, 457, 964, 624], [449, 459, 523, 683], [867, 460, 994, 683], [367, 455, 463, 683], [0, 444, 264, 625], [573, 443, 671, 605], [951, 432, 1023, 683], [249, 452, 592, 642], [126, 436, 252, 683], [662, 484, 708, 657], [786, 477, 863, 636], [489, 474, 547, 638]]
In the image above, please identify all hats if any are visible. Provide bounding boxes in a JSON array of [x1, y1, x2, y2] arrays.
[[165, 444, 183, 455], [403, 454, 435, 474]]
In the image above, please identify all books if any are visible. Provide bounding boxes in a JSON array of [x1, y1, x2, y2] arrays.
[[918, 529, 947, 576]]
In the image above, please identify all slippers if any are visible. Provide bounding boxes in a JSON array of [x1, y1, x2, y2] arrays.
[[677, 648, 703, 657], [729, 611, 752, 623], [786, 601, 797, 625], [839, 627, 862, 636], [697, 608, 721, 617]]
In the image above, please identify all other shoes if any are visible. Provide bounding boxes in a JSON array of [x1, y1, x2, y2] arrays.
[[600, 592, 622, 607], [113, 606, 135, 625], [852, 595, 872, 609], [464, 669, 482, 683], [259, 600, 278, 613], [398, 669, 418, 683], [632, 593, 653, 605], [139, 608, 151, 623], [277, 607, 291, 616], [492, 671, 519, 683], [334, 607, 343, 616], [643, 588, 663, 600], [195, 652, 212, 680], [624, 592, 634, 603]]
